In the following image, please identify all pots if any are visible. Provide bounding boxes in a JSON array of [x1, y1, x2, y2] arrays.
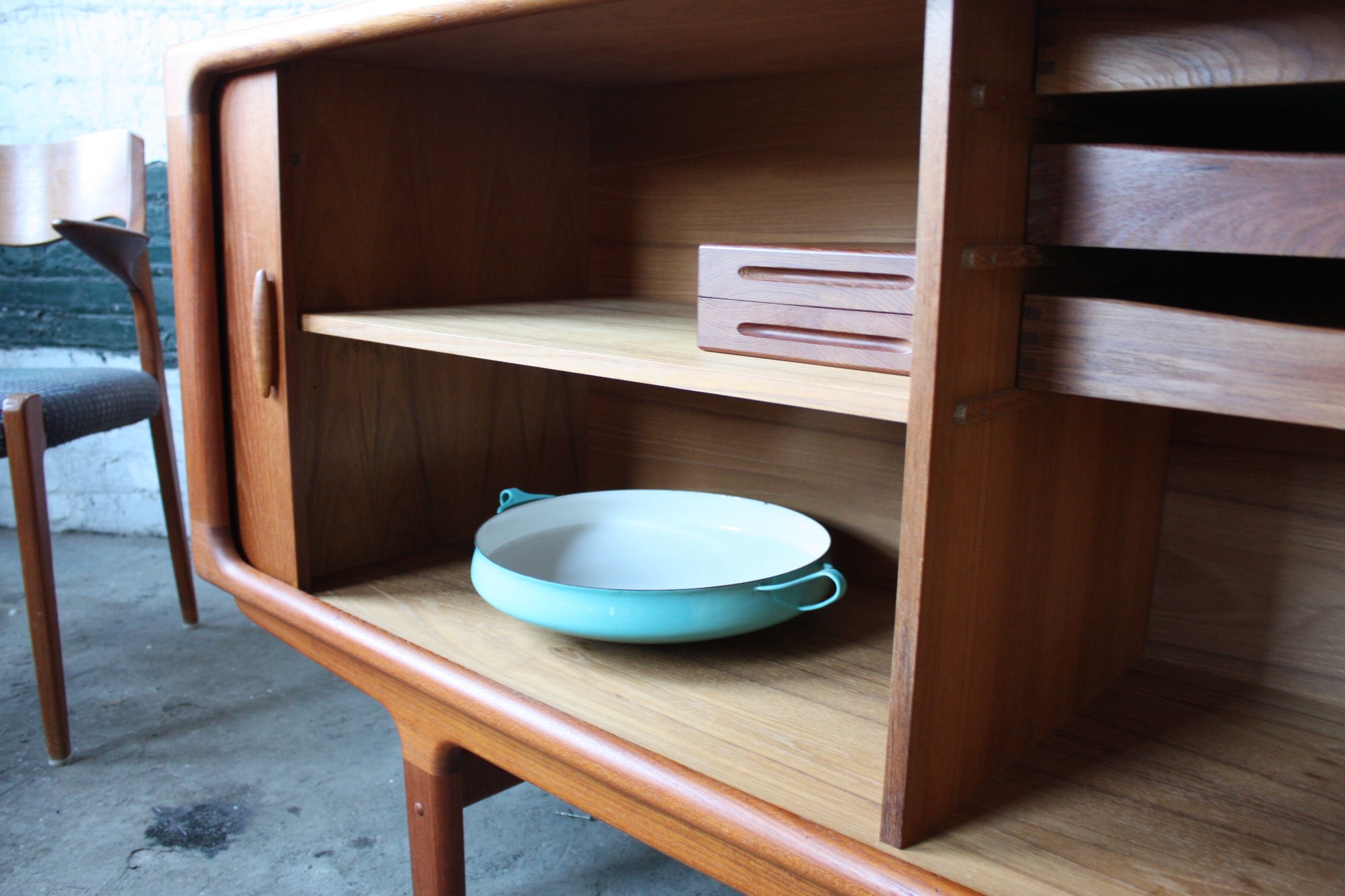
[[470, 486, 847, 643]]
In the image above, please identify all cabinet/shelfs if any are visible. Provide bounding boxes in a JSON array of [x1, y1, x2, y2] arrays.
[[166, 0, 1345, 896]]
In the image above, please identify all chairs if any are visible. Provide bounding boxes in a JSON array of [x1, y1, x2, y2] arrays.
[[0, 135, 202, 766]]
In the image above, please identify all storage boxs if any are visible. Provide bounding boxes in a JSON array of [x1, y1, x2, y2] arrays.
[[697, 246, 915, 375]]
